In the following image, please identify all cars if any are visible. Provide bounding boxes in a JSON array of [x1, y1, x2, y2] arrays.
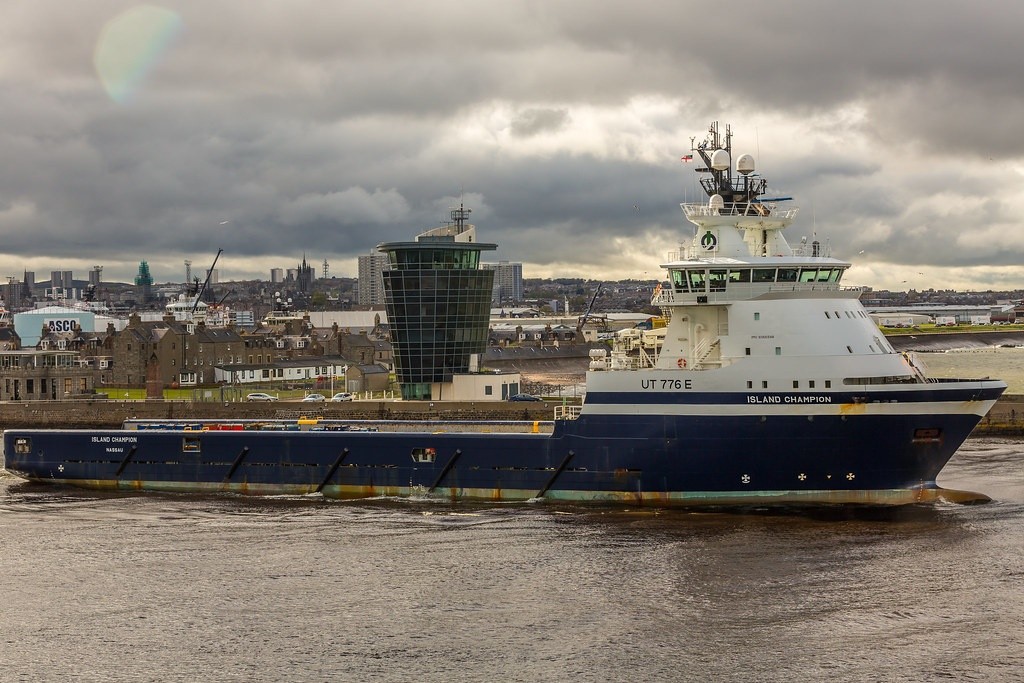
[[508, 392, 541, 402], [246, 393, 279, 403], [330, 392, 353, 402], [302, 393, 325, 403]]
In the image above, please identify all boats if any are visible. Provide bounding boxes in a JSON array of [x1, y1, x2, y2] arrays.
[[0, 116, 1009, 508]]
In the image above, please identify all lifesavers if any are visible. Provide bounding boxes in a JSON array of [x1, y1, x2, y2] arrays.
[[678, 359, 686, 368]]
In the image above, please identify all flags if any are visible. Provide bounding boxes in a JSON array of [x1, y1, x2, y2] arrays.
[[680, 154, 692, 163]]
